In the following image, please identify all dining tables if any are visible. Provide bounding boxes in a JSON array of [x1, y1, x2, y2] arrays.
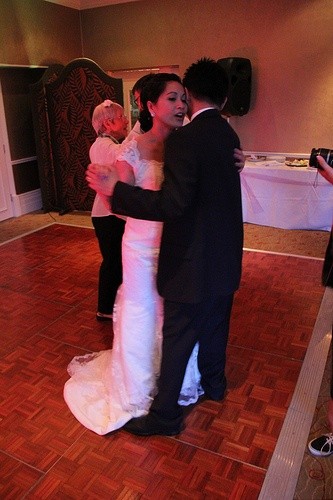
[[240, 160, 333, 232]]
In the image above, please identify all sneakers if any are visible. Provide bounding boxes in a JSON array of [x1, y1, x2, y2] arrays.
[[307, 433, 333, 456]]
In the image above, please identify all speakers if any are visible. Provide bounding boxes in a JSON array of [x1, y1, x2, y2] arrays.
[[217, 57, 252, 117]]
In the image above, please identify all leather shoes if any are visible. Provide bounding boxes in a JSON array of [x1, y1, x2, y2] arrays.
[[123, 418, 183, 436], [203, 388, 224, 400]]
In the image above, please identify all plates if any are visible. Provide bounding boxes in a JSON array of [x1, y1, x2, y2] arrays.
[[285, 160, 309, 166], [246, 157, 265, 162]]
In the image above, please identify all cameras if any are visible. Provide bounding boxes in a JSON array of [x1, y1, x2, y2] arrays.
[[309, 148, 333, 170]]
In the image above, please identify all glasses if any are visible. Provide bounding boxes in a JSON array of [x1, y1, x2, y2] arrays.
[[133, 96, 140, 106]]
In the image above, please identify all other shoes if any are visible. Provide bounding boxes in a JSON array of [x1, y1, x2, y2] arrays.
[[95, 313, 112, 320]]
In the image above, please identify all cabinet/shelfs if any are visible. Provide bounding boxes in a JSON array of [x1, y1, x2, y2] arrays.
[[0, 64, 49, 218]]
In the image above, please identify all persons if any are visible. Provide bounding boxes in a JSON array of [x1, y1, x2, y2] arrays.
[[89, 73, 192, 320], [86, 56, 244, 435], [308, 156, 333, 457], [89, 72, 245, 415]]
